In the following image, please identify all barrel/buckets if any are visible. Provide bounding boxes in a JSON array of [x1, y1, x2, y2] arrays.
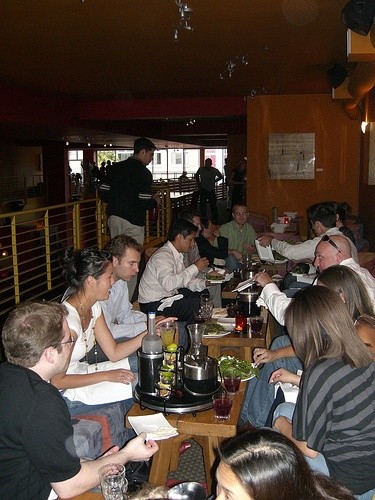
[[239, 268, 261, 317], [136, 347, 164, 396], [183, 355, 222, 397]]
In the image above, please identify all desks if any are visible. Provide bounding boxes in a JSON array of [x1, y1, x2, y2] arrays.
[[202, 316, 267, 363], [256, 260, 288, 271], [124, 380, 249, 496], [222, 277, 282, 299]]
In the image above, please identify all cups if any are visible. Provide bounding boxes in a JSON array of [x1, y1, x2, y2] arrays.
[[200, 294, 215, 317], [235, 316, 248, 333], [250, 316, 264, 334], [211, 393, 234, 423], [98, 462, 125, 500], [242, 254, 252, 268], [157, 320, 175, 348], [222, 370, 241, 395], [227, 296, 240, 318]]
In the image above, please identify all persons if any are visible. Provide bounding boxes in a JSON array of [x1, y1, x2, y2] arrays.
[[240, 202, 375, 431], [272, 285, 375, 496], [195, 158, 223, 218], [98, 138, 161, 246], [218, 203, 258, 260], [224, 158, 230, 187], [178, 171, 188, 181], [231, 159, 247, 206], [81, 234, 168, 365], [0, 301, 160, 500], [138, 217, 216, 322], [60, 250, 177, 417], [92, 161, 115, 185], [178, 213, 242, 269], [214, 427, 359, 500]]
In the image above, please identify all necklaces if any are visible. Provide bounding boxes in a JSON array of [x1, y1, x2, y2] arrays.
[[76, 292, 98, 374]]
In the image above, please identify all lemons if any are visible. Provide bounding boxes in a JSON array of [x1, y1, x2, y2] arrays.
[[160, 365, 175, 382], [166, 343, 177, 352], [208, 331, 217, 335]]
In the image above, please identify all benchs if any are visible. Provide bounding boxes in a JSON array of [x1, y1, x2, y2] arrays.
[[59, 319, 189, 465]]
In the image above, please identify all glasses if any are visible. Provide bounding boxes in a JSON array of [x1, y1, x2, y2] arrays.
[[321, 235, 341, 253], [43, 336, 75, 349]]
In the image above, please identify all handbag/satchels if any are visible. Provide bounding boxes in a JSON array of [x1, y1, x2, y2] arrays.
[[118, 439, 152, 494]]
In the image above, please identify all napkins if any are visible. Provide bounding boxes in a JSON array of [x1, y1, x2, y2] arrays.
[[126, 412, 179, 442]]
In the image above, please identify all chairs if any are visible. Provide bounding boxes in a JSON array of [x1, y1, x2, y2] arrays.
[[249, 212, 269, 233]]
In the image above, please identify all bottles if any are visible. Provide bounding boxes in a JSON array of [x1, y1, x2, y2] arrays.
[[272, 207, 278, 223], [285, 218, 289, 224], [186, 323, 207, 357], [141, 311, 162, 353], [174, 320, 188, 351]]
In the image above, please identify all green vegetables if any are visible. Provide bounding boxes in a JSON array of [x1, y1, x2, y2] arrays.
[[206, 274, 225, 280], [217, 353, 260, 379], [203, 322, 225, 335]]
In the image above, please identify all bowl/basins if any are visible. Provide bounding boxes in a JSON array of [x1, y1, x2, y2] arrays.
[[217, 317, 250, 331]]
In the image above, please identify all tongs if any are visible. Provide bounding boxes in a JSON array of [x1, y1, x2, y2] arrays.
[[189, 354, 208, 370]]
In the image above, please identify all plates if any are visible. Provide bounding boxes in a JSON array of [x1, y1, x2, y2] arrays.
[[218, 363, 257, 381], [203, 332, 232, 338], [205, 266, 226, 284], [239, 253, 289, 265], [128, 413, 179, 442], [212, 308, 228, 318]]
